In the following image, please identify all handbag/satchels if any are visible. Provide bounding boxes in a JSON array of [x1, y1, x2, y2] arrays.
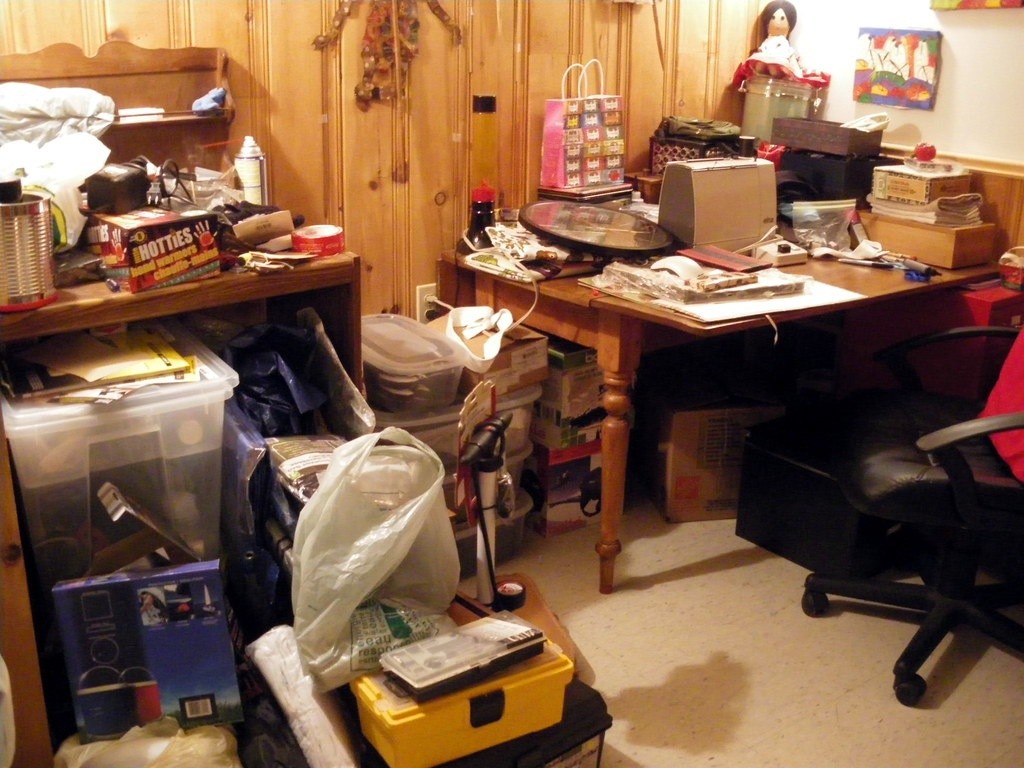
[[540, 59, 626, 189], [655, 116, 741, 154], [291, 426, 462, 693]]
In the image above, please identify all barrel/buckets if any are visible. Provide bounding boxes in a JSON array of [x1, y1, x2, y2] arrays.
[[738, 76, 820, 149], [0, 192, 57, 312]]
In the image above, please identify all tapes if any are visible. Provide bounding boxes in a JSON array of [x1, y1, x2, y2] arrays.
[[495, 580, 526, 611], [292, 224, 344, 258]]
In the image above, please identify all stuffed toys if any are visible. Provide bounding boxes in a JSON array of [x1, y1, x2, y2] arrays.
[[747, 0, 803, 79]]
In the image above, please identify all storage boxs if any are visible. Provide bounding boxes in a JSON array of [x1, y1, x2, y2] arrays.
[[872, 165, 977, 207], [342, 636, 575, 768], [77, 203, 219, 296], [0, 314, 238, 592], [768, 117, 885, 159], [50, 558, 244, 746], [849, 211, 995, 269], [641, 375, 787, 522], [355, 313, 609, 586]]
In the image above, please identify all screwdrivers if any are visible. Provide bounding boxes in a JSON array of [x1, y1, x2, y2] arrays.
[[102, 276, 122, 293]]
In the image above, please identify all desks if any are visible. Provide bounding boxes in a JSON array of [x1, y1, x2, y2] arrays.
[[444, 233, 1005, 591], [0, 246, 366, 747]]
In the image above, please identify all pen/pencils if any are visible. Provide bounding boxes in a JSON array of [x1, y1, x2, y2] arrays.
[[837, 259, 894, 268]]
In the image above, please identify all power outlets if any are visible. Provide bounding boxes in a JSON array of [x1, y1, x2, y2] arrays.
[[416, 284, 436, 326]]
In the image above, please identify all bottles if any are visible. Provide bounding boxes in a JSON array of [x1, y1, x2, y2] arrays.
[[458, 186, 496, 255], [234, 135, 268, 207]]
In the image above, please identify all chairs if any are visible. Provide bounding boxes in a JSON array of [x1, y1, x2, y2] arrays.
[[799, 324, 1024, 707]]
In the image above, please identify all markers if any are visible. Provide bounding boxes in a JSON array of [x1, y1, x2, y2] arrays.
[[904, 259, 931, 276]]
